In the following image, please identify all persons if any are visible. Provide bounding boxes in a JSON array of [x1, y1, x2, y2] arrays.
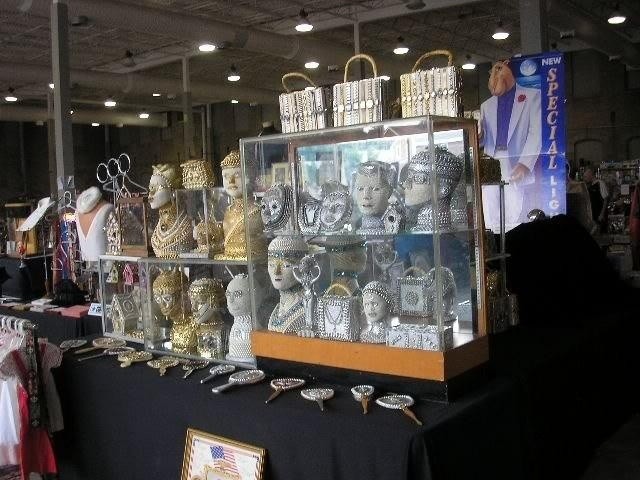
[[476, 60, 542, 234], [147, 144, 464, 365], [583, 169, 609, 236], [73, 185, 114, 262]]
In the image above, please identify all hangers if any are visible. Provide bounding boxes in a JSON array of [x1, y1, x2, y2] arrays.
[[1, 313, 45, 355]]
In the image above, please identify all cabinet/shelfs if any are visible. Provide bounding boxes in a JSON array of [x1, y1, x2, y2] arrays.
[[96, 185, 255, 367], [238, 119, 488, 384], [594, 161, 640, 273]]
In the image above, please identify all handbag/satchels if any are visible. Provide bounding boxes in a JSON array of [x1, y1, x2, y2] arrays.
[[333, 54, 393, 126], [278, 73, 331, 134], [397, 267, 434, 317], [317, 284, 361, 342], [400, 50, 464, 118]]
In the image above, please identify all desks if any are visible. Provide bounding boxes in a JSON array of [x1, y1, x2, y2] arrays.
[[46, 332, 507, 479]]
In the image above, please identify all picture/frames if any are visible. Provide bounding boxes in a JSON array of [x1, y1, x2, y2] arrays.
[[179, 424, 268, 480]]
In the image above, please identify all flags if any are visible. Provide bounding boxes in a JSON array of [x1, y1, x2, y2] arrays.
[[209, 445, 239, 475]]
